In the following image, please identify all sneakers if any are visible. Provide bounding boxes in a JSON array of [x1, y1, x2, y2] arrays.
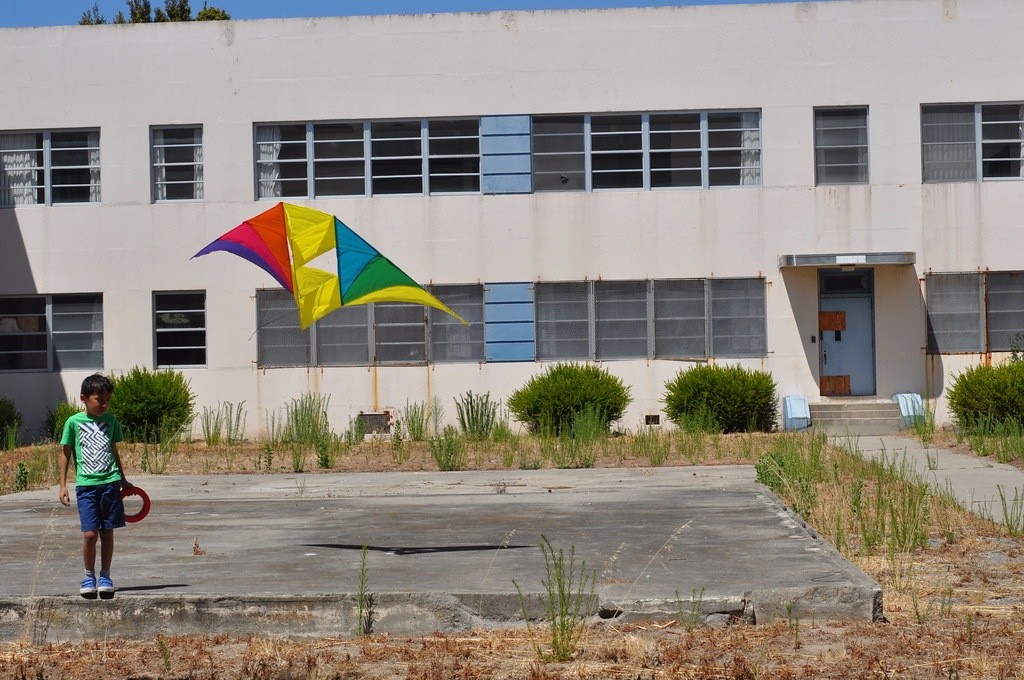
[[80, 577, 97, 594], [98, 576, 114, 593]]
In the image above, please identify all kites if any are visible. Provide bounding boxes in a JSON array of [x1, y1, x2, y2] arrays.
[[189, 202, 471, 331]]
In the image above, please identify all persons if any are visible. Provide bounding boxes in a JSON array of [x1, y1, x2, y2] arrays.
[[59, 375, 137, 593]]
